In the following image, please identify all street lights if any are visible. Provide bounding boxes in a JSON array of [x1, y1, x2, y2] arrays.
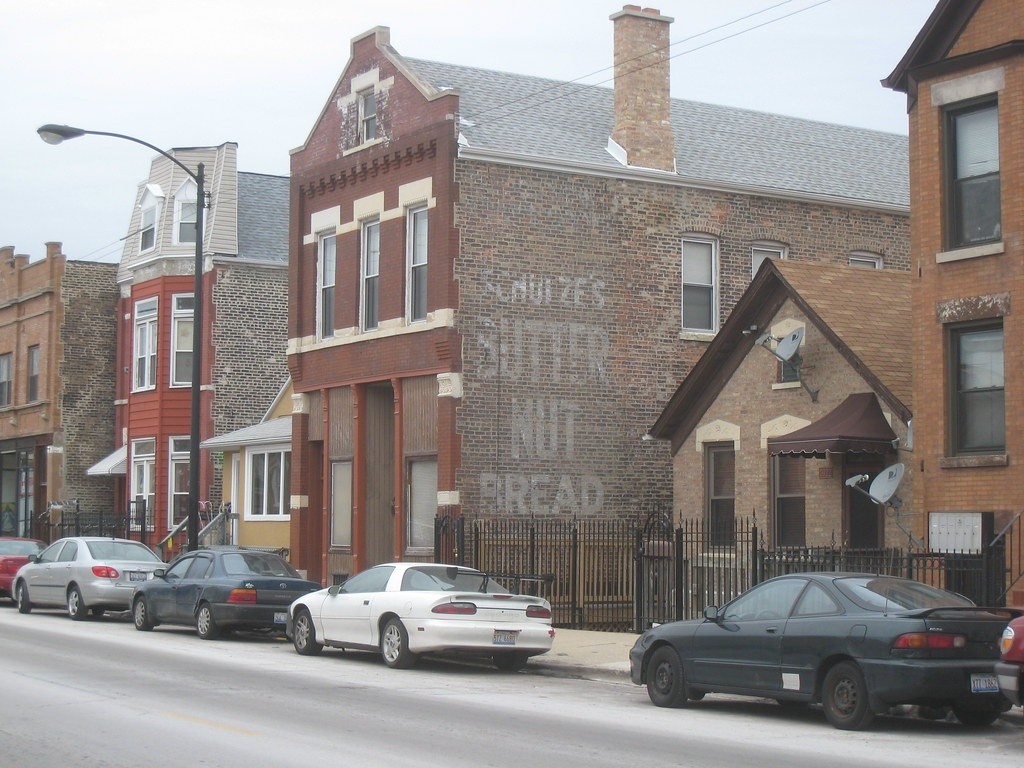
[[37, 124, 205, 551]]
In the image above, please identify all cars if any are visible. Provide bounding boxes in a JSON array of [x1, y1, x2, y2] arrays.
[[130, 549, 321, 641], [286, 562, 557, 668], [11, 538, 171, 623], [0, 536, 50, 597], [995, 614, 1024, 712], [629, 572, 1024, 733]]
